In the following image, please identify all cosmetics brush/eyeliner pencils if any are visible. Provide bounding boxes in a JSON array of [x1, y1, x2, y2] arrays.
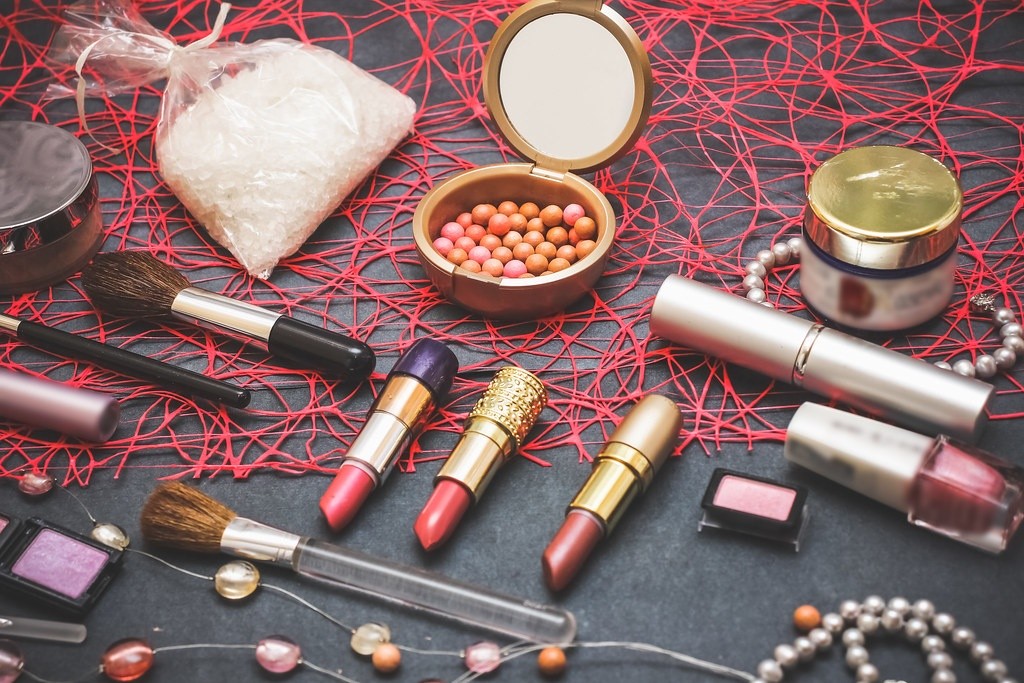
[[0, 306, 259, 409], [139, 477, 580, 653], [80, 249, 377, 382]]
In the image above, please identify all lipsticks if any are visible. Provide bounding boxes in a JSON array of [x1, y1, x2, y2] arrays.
[[318, 335, 462, 536], [541, 392, 684, 599], [648, 271, 1000, 451], [0, 363, 120, 440], [415, 365, 550, 554]]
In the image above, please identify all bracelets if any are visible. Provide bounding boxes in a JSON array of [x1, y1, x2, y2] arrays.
[[742, 232, 1023, 380]]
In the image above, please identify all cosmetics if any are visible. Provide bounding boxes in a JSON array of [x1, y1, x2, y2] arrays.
[[0, 510, 127, 623], [782, 398, 1023, 560], [797, 144, 966, 343], [410, 0, 657, 319], [695, 466, 817, 555], [0, 116, 106, 297]]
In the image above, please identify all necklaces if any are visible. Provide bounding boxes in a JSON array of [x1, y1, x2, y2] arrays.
[[0, 457, 1024, 683]]
